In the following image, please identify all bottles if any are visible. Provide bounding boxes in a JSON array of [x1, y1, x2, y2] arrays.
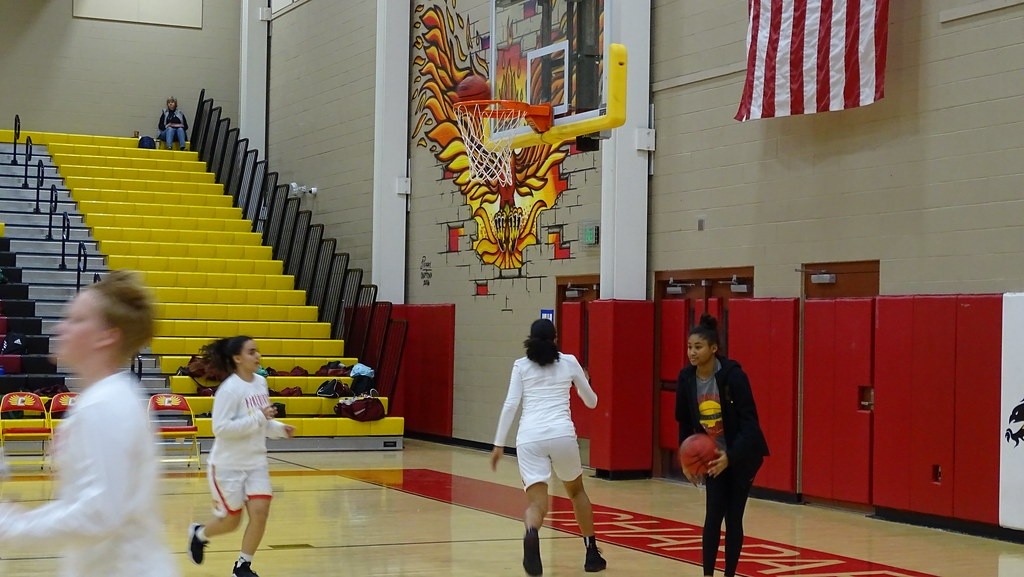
[[0, 365, 6, 375]]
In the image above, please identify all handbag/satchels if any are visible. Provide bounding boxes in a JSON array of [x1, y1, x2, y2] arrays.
[[336, 403, 353, 416], [316, 380, 341, 398], [353, 400, 384, 422]]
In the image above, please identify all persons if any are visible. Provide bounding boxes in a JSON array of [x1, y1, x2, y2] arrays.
[[0, 270, 185, 577], [187, 336, 296, 577], [674, 314, 771, 577], [156, 96, 189, 152], [490, 319, 607, 577]]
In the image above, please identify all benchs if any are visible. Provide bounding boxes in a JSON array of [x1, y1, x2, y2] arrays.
[[0, 130, 405, 439]]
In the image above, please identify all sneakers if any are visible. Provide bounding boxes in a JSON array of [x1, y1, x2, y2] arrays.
[[585, 555, 608, 572], [187, 524, 206, 564], [524, 533, 544, 576], [233, 561, 259, 577]]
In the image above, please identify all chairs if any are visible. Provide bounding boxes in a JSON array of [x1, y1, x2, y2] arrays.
[[145, 393, 203, 474], [47, 392, 81, 472], [0, 392, 55, 473]]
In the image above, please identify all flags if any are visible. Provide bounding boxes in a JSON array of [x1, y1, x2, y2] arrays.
[[732, 0, 890, 121]]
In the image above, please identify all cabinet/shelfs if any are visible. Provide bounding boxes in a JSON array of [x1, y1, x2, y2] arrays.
[[725, 296, 803, 500], [871, 293, 1024, 531], [799, 297, 877, 515]]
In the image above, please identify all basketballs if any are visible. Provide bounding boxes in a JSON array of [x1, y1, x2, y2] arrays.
[[677, 434, 721, 480], [456, 75, 493, 115]]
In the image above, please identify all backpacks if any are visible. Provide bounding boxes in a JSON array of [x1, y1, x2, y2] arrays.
[[350, 376, 373, 396], [138, 136, 154, 149], [1, 331, 28, 355]]
